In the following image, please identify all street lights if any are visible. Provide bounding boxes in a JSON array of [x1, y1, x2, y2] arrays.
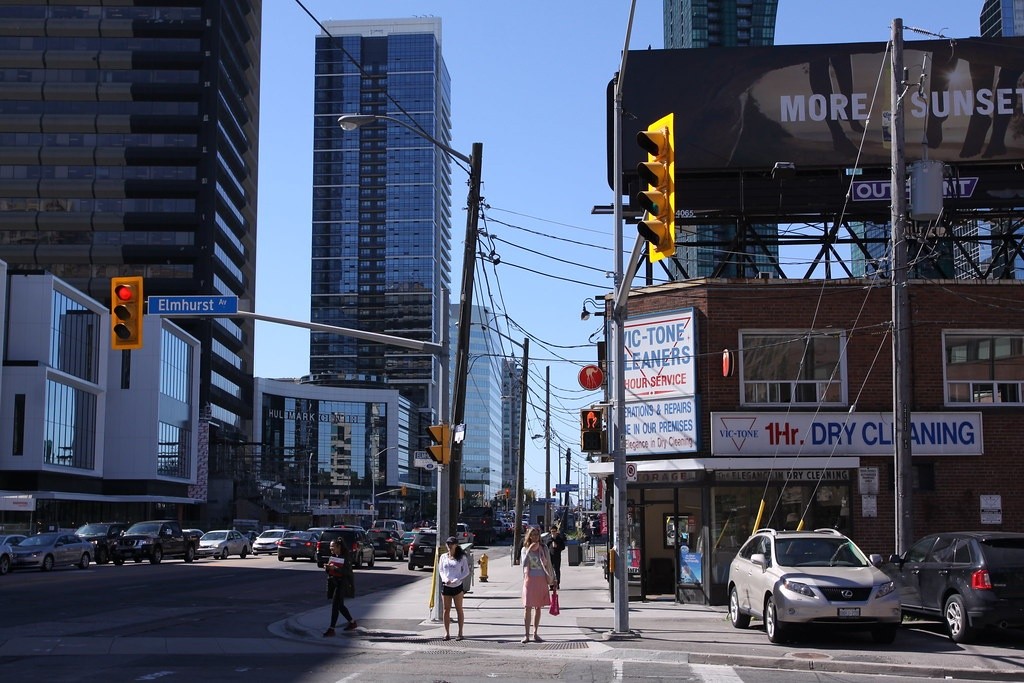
[[338, 112, 485, 625], [370, 446, 396, 522]]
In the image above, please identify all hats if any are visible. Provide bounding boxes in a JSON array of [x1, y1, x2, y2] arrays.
[[446, 537, 458, 544]]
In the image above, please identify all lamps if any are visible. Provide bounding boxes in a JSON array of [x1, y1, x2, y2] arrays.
[[581, 298, 607, 319], [772, 161, 794, 205]]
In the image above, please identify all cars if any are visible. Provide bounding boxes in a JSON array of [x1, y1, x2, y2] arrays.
[[552, 506, 601, 536], [251, 529, 290, 556], [195, 529, 250, 560], [878, 529, 1024, 644], [0, 534, 28, 576], [307, 527, 329, 540], [239, 530, 260, 552], [399, 532, 418, 557], [11, 531, 95, 572], [277, 531, 320, 563], [167, 529, 205, 560]]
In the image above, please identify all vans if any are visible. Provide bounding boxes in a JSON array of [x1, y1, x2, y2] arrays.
[[372, 519, 402, 532], [411, 506, 530, 549]]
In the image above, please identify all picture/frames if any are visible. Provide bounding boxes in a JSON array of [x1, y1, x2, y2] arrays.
[[663, 513, 692, 549]]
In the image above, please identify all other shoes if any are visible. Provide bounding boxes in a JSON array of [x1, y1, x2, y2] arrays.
[[533, 635, 543, 643], [323, 628, 337, 637], [550, 586, 554, 590], [442, 635, 451, 640], [557, 584, 560, 589], [522, 637, 530, 643], [344, 621, 358, 630], [456, 635, 464, 641]]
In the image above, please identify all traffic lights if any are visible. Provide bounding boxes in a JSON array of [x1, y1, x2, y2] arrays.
[[424, 425, 450, 465], [506, 489, 509, 498], [552, 488, 556, 495], [580, 409, 603, 452], [110, 273, 144, 352], [633, 113, 677, 262]]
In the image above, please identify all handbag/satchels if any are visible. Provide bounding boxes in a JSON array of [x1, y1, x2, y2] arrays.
[[550, 585, 559, 616]]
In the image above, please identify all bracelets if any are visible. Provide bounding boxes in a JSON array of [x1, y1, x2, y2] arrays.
[[554, 580, 557, 581]]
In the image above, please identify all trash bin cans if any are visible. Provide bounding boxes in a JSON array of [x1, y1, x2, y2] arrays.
[[568, 545, 582, 566], [460, 543, 474, 594]]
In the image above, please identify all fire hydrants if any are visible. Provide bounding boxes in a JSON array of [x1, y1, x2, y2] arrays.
[[476, 553, 489, 582]]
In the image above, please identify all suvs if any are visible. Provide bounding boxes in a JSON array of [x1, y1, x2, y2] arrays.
[[363, 528, 406, 561], [74, 522, 132, 564], [726, 527, 903, 644], [407, 530, 436, 570], [316, 524, 375, 569]]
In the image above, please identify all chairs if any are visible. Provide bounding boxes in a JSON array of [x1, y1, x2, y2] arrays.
[[816, 543, 831, 560], [775, 543, 793, 566]]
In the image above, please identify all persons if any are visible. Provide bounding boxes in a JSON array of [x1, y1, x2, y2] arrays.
[[808, 52, 1024, 158], [582, 518, 588, 530], [521, 528, 558, 644], [322, 537, 357, 637], [546, 526, 565, 590], [439, 537, 469, 641]]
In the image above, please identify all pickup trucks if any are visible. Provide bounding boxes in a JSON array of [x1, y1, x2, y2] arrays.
[[111, 519, 200, 564]]
